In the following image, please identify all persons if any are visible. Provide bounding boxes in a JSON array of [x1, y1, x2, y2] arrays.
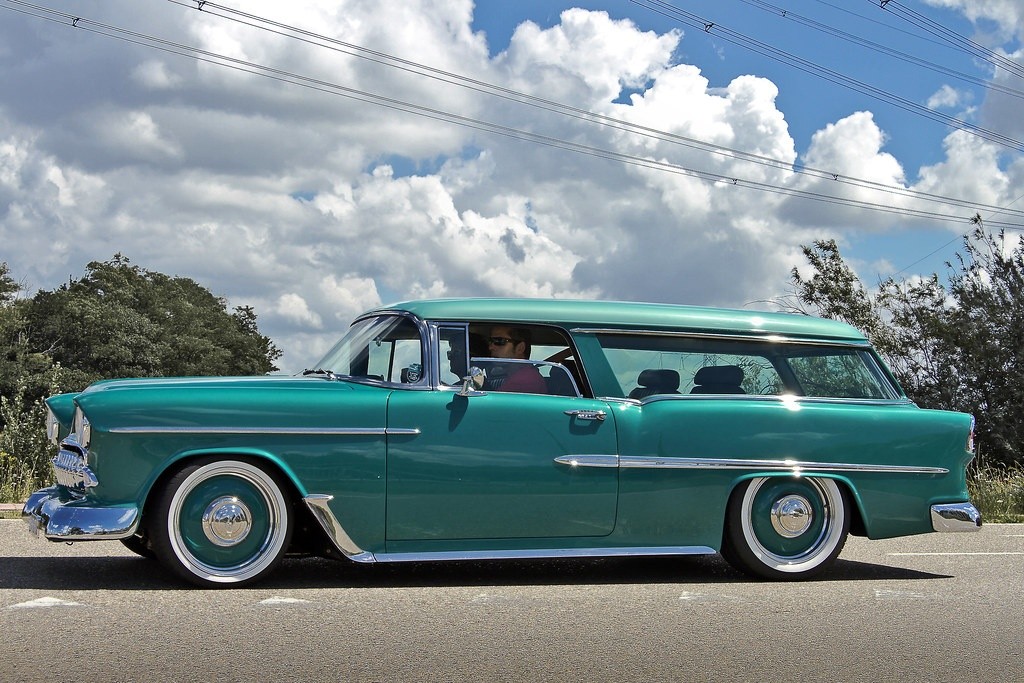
[[447, 323, 550, 394]]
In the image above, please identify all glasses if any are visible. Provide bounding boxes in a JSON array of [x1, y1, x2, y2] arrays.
[[487, 337, 519, 347], [447, 351, 477, 360]]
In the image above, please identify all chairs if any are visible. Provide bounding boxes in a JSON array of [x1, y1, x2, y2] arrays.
[[549, 360, 586, 396], [691, 366, 745, 393], [627, 369, 682, 400]]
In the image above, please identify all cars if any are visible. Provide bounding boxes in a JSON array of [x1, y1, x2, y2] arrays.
[[16, 296, 984, 590]]
